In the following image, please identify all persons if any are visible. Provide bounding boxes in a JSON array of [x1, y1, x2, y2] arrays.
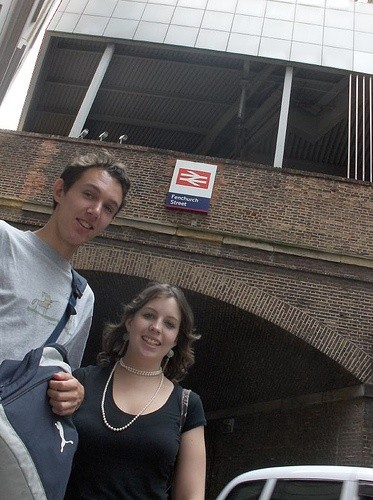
[[0, 149, 131, 416], [70, 284, 206, 500]]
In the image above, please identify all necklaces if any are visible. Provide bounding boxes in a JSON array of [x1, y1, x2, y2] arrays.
[[101, 357, 164, 431]]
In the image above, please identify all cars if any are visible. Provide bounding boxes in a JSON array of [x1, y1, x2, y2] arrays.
[[215, 465, 373, 500]]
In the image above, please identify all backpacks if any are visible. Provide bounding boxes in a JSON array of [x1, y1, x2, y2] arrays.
[[0, 269, 88, 500]]
